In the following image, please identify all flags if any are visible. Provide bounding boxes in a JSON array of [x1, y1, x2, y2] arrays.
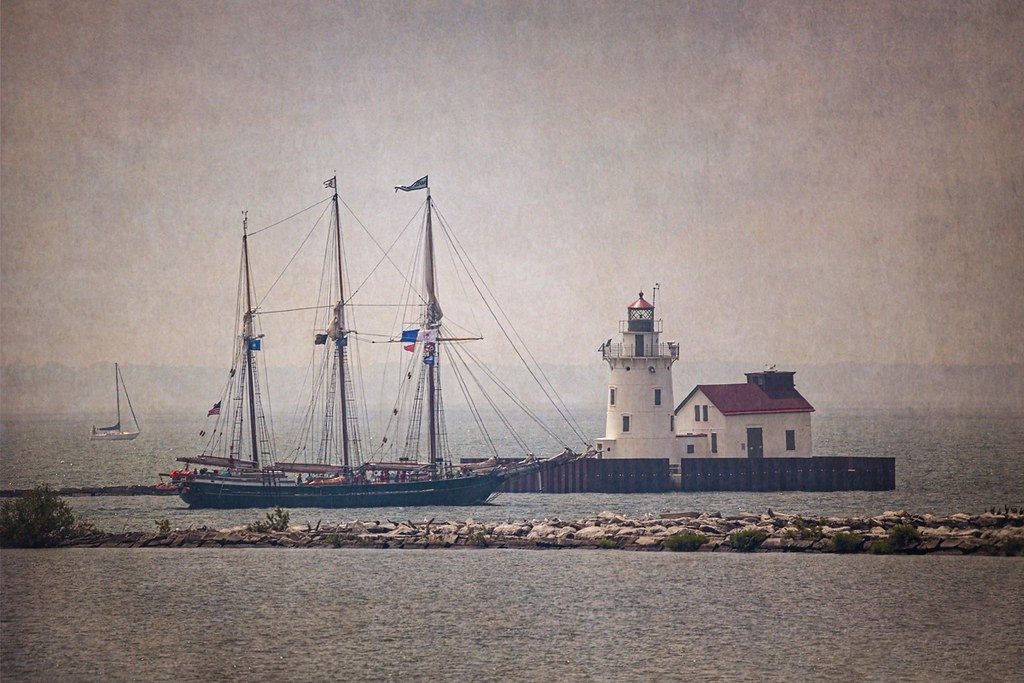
[[323, 177, 337, 188], [207, 400, 221, 416], [395, 175, 429, 192]]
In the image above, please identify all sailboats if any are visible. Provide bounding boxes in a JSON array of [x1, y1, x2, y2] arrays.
[[157, 187, 605, 509], [89, 363, 140, 440]]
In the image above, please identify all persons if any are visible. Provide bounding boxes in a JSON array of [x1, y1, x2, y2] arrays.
[[454, 465, 475, 478], [374, 469, 410, 482], [296, 473, 313, 486]]
